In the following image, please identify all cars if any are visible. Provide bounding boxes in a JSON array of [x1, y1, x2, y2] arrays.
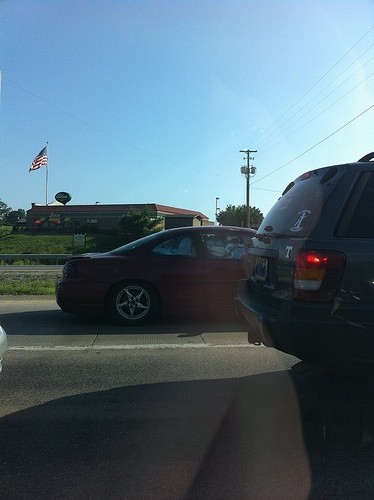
[[55, 224, 259, 327]]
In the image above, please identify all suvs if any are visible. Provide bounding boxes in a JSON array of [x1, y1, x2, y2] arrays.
[[231, 149, 374, 377]]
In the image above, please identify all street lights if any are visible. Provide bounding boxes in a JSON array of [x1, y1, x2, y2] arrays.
[[238, 150, 258, 229]]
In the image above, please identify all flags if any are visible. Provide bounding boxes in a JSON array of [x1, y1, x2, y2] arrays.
[[29, 145, 48, 173]]
